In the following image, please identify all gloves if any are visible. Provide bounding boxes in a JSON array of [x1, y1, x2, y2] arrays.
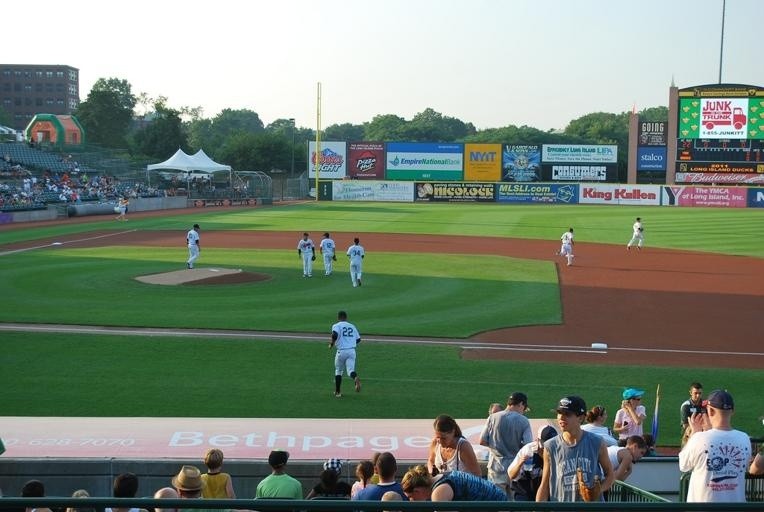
[[299, 255, 301, 259]]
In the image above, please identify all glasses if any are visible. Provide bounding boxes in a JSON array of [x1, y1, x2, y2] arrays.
[[630, 399, 641, 401], [598, 405, 603, 416], [443, 461, 447, 473]]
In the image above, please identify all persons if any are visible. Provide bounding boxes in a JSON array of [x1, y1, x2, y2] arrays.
[[679, 390, 752, 503], [351, 460, 374, 500], [536, 397, 614, 502], [67, 489, 95, 512], [201, 449, 236, 499], [370, 452, 382, 484], [304, 459, 352, 500], [105, 473, 148, 512], [508, 425, 558, 502], [613, 389, 647, 447], [680, 383, 706, 447], [256, 449, 303, 500], [320, 233, 337, 276], [401, 466, 507, 512], [428, 415, 482, 477], [172, 465, 258, 512], [381, 492, 403, 512], [297, 233, 315, 278], [479, 392, 533, 501], [580, 406, 612, 436], [328, 311, 361, 398], [21, 480, 52, 512], [484, 403, 505, 460], [186, 224, 200, 270], [347, 238, 364, 288], [0, 157, 210, 222], [749, 448, 764, 475], [558, 228, 574, 266], [627, 217, 644, 252], [153, 488, 178, 512], [599, 435, 648, 484], [352, 452, 410, 512]]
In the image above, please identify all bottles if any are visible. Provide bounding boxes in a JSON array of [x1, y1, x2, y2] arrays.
[[524, 446, 533, 471], [431, 464, 440, 476]]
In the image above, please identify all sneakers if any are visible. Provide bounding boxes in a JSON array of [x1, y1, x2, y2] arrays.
[[334, 392, 341, 398], [567, 264, 572, 267], [187, 262, 189, 268], [355, 376, 361, 392], [324, 271, 332, 276], [308, 275, 312, 278], [627, 246, 630, 251], [189, 268, 194, 269], [637, 246, 640, 252], [358, 277, 361, 286], [303, 275, 308, 277], [556, 250, 560, 257], [353, 285, 357, 287]]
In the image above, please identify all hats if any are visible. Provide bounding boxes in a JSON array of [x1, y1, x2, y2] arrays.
[[354, 238, 359, 242], [338, 311, 347, 318], [541, 427, 558, 446], [171, 465, 207, 491], [557, 396, 587, 415], [702, 390, 734, 410], [333, 255, 337, 261], [637, 218, 641, 220], [323, 458, 342, 477], [193, 224, 200, 229], [269, 451, 290, 468], [72, 190, 75, 192], [570, 228, 573, 232], [509, 392, 531, 412], [323, 233, 329, 237], [699, 400, 708, 408], [622, 389, 645, 400], [642, 434, 655, 451], [304, 232, 309, 236], [312, 256, 316, 261]]
